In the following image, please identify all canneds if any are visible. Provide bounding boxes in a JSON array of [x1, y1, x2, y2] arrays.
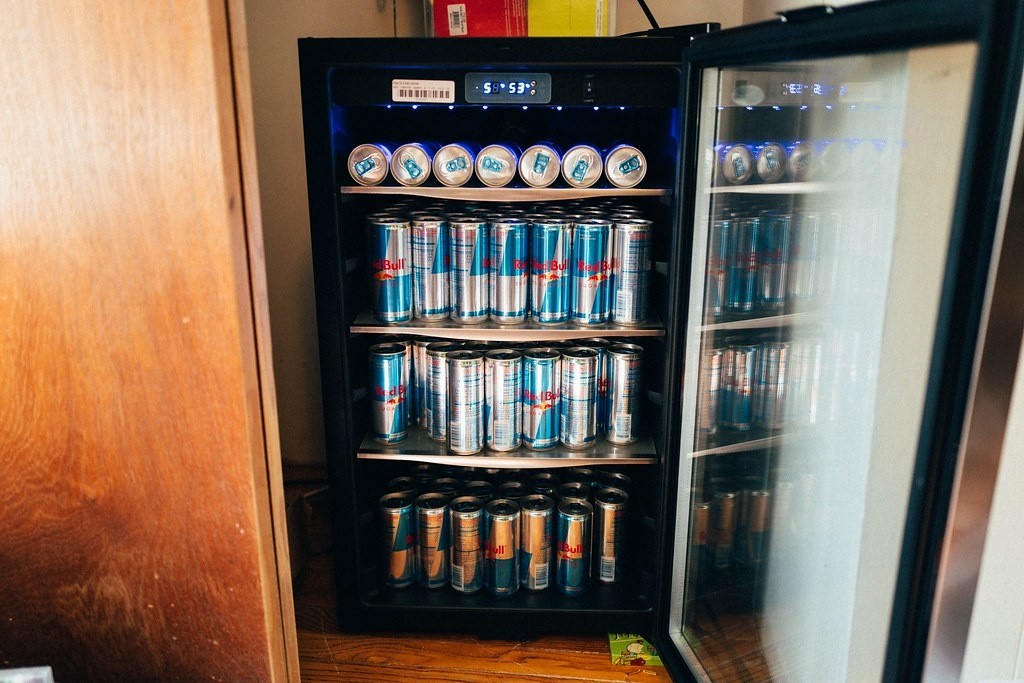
[[365, 333, 644, 456], [689, 140, 878, 585], [363, 199, 654, 327], [348, 139, 647, 188], [375, 463, 633, 598]]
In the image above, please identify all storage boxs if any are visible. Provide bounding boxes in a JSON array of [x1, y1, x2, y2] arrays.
[[527, 0, 609, 37], [432, 0, 528, 37]]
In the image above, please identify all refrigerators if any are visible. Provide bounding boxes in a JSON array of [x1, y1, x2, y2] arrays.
[[298, 0, 1024, 683]]
